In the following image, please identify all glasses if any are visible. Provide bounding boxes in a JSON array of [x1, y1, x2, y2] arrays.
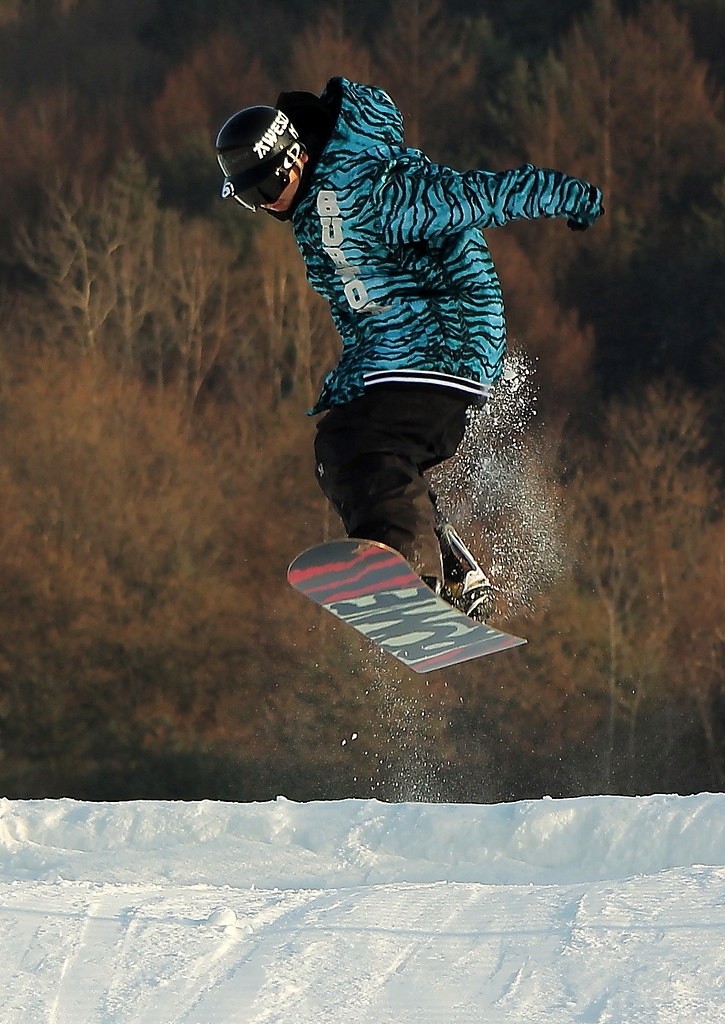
[[233, 143, 305, 212]]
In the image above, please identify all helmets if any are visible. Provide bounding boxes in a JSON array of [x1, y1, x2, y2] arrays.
[[215, 106, 298, 177]]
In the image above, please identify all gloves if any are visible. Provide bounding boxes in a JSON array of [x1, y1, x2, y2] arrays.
[[567, 205, 604, 230]]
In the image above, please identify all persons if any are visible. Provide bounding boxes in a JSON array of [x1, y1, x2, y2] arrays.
[[212, 75, 606, 617]]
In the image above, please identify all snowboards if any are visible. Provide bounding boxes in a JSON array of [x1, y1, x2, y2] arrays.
[[285, 536, 530, 675]]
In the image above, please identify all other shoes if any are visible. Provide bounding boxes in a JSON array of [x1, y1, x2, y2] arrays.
[[442, 568, 497, 624], [421, 576, 442, 596]]
